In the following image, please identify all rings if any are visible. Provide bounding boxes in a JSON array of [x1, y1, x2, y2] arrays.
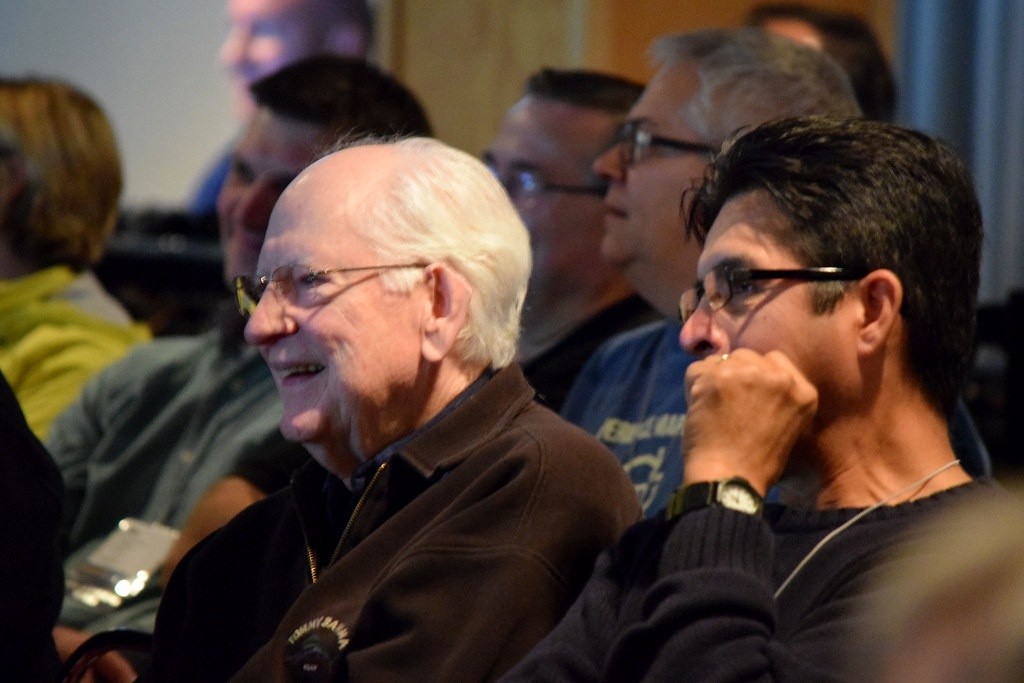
[[717, 354, 730, 364]]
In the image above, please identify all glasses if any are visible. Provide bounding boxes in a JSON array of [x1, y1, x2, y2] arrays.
[[231, 263, 431, 317], [678, 261, 868, 320], [481, 151, 607, 199], [614, 117, 721, 163]]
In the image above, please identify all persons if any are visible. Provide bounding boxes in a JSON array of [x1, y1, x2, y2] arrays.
[[0, 73, 161, 441], [745, 3, 904, 143], [188, 3, 383, 215], [474, 63, 659, 419], [488, 115, 982, 682], [37, 54, 442, 683], [551, 24, 861, 522], [119, 136, 645, 683]]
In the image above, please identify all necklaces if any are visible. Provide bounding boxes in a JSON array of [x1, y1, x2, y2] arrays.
[[770, 457, 969, 602]]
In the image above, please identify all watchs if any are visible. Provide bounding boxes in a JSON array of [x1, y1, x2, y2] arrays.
[[663, 475, 773, 534]]
